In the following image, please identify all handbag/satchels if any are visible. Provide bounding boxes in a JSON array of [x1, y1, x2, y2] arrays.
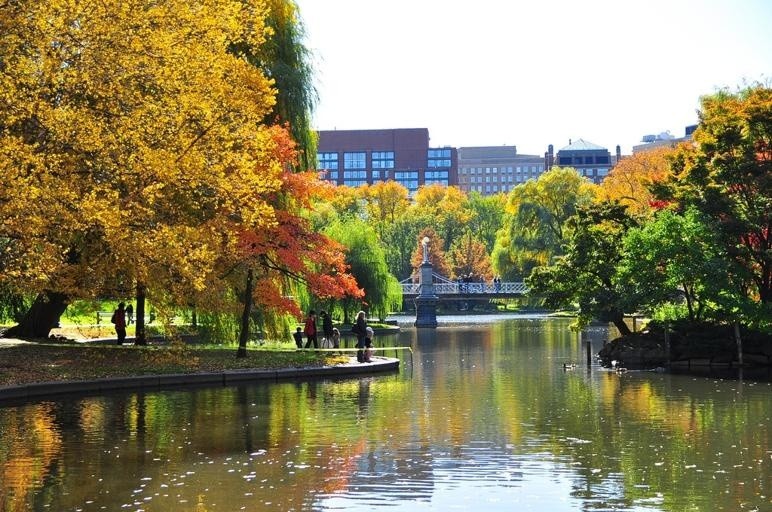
[[352, 325, 360, 333], [321, 335, 333, 348]]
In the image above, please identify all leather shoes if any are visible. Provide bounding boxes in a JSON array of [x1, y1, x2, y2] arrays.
[[358, 359, 373, 362]]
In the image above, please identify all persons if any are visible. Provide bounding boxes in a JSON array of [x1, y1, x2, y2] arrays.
[[304, 311, 320, 348], [353, 311, 375, 363], [125, 303, 136, 326], [111, 302, 126, 345], [332, 329, 343, 356], [293, 327, 304, 348], [319, 310, 334, 339]]
